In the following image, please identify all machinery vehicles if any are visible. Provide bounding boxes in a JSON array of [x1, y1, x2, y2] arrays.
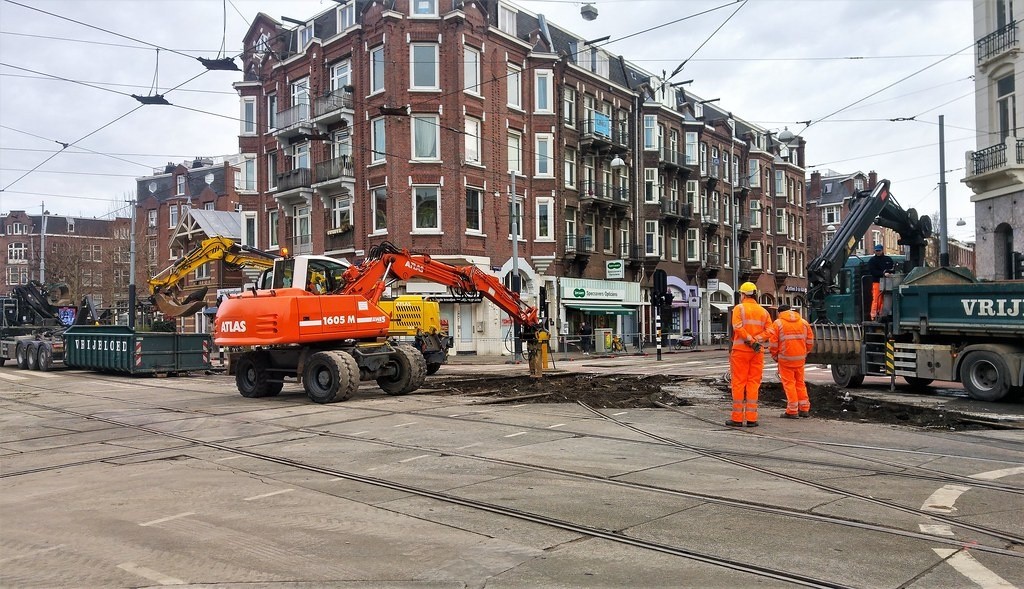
[[806, 178, 1024, 402], [146, 236, 454, 375], [214, 241, 550, 405]]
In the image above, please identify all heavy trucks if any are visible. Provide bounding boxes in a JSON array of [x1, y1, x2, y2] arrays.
[[0, 283, 100, 371]]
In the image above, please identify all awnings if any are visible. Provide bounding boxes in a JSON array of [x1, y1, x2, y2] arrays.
[[710, 303, 773, 313], [564, 303, 636, 316]]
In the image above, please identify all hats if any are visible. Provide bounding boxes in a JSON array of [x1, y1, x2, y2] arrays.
[[874, 244, 883, 251], [777, 304, 792, 312]]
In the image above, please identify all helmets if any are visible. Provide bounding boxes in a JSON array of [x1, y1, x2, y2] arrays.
[[739, 282, 758, 293]]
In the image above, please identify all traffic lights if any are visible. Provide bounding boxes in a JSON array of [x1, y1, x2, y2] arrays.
[[660, 294, 672, 305]]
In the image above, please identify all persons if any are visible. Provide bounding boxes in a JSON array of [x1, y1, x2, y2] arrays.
[[865, 244, 894, 322], [767, 306, 814, 420], [578, 321, 592, 355], [725, 282, 775, 427]]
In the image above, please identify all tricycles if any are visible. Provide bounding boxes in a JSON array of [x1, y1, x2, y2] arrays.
[[675, 337, 695, 349]]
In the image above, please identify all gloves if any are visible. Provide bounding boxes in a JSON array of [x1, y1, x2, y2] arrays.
[[745, 340, 763, 353]]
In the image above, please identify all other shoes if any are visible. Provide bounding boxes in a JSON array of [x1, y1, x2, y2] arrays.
[[798, 411, 809, 417], [726, 420, 743, 427], [747, 421, 759, 426], [780, 412, 799, 420]]
